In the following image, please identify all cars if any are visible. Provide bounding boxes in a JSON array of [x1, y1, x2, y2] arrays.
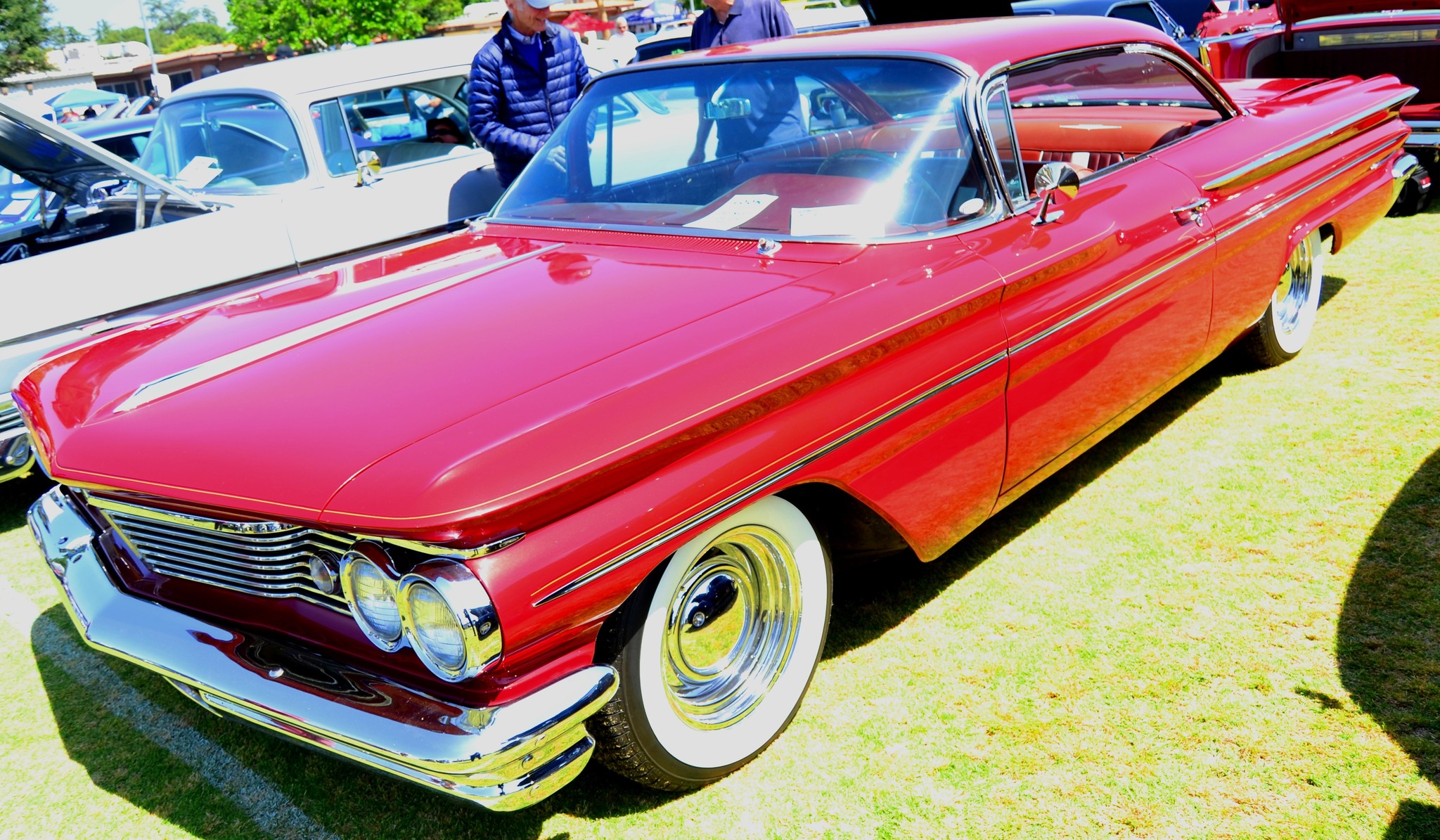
[[0, 1, 1439, 507], [13, 10, 1424, 824]]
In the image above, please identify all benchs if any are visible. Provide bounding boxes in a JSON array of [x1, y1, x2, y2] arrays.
[[730, 118, 1193, 228]]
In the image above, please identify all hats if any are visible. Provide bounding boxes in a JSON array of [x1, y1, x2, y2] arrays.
[[274, 46, 293, 57], [526, 0, 565, 9]]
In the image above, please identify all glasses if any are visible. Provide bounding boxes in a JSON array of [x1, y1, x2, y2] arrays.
[[429, 128, 456, 136]]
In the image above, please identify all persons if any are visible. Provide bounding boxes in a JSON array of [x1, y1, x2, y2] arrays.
[[686, 13, 697, 26], [688, 0, 809, 167], [84, 105, 96, 120], [149, 90, 165, 111], [606, 17, 639, 67], [468, 0, 596, 196]]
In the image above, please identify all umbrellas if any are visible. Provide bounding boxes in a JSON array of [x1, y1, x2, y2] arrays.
[[561, 11, 615, 42]]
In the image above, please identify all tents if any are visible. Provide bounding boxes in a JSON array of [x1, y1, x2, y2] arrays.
[[45, 86, 130, 121]]
